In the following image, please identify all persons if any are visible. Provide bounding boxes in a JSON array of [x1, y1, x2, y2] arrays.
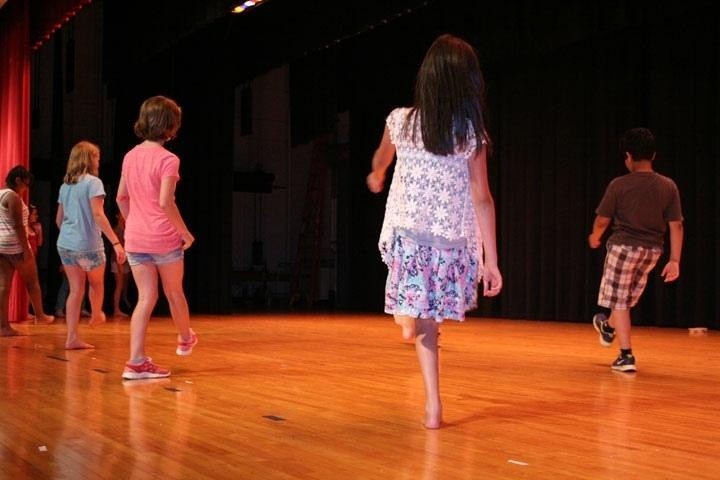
[[52, 139, 127, 350], [365, 26, 507, 433], [114, 93, 202, 381], [18, 203, 44, 325], [111, 211, 130, 318], [57, 268, 96, 319], [580, 124, 686, 372], [0, 164, 58, 340]]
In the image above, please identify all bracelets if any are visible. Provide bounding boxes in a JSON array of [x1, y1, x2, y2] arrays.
[[112, 241, 122, 247]]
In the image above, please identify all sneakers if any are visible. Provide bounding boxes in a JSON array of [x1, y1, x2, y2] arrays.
[[176, 327, 198, 357], [122, 357, 170, 380], [593, 313, 616, 348], [611, 350, 637, 372]]
